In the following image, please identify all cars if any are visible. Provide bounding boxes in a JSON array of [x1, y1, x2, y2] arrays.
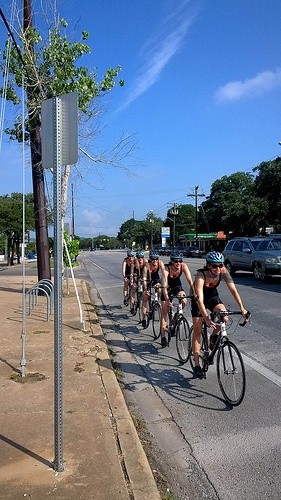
[[153, 246, 203, 258]]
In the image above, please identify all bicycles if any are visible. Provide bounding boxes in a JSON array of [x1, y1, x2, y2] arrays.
[[125, 274, 199, 363], [187, 309, 252, 406]]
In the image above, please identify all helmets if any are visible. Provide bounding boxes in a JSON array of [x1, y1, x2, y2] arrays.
[[149, 250, 160, 257], [170, 251, 183, 260], [136, 251, 144, 257], [206, 250, 224, 263], [127, 250, 134, 256]]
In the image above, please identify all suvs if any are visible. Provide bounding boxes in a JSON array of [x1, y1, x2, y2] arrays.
[[221, 237, 281, 283]]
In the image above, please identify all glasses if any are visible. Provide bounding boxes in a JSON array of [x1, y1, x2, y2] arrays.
[[173, 259, 183, 263], [150, 257, 159, 260], [128, 255, 133, 257], [211, 264, 222, 268], [137, 256, 143, 259]]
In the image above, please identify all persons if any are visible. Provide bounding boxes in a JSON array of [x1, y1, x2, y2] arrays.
[[190, 251, 251, 377], [122, 250, 164, 327], [161, 252, 193, 346]]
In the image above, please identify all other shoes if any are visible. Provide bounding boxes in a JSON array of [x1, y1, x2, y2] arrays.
[[150, 313, 153, 319], [131, 308, 135, 314], [161, 337, 168, 346], [193, 366, 203, 377], [124, 300, 127, 305], [210, 334, 217, 350], [170, 328, 176, 335], [142, 320, 146, 327]]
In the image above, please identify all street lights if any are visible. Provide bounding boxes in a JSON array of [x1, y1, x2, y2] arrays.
[[165, 217, 175, 248]]
